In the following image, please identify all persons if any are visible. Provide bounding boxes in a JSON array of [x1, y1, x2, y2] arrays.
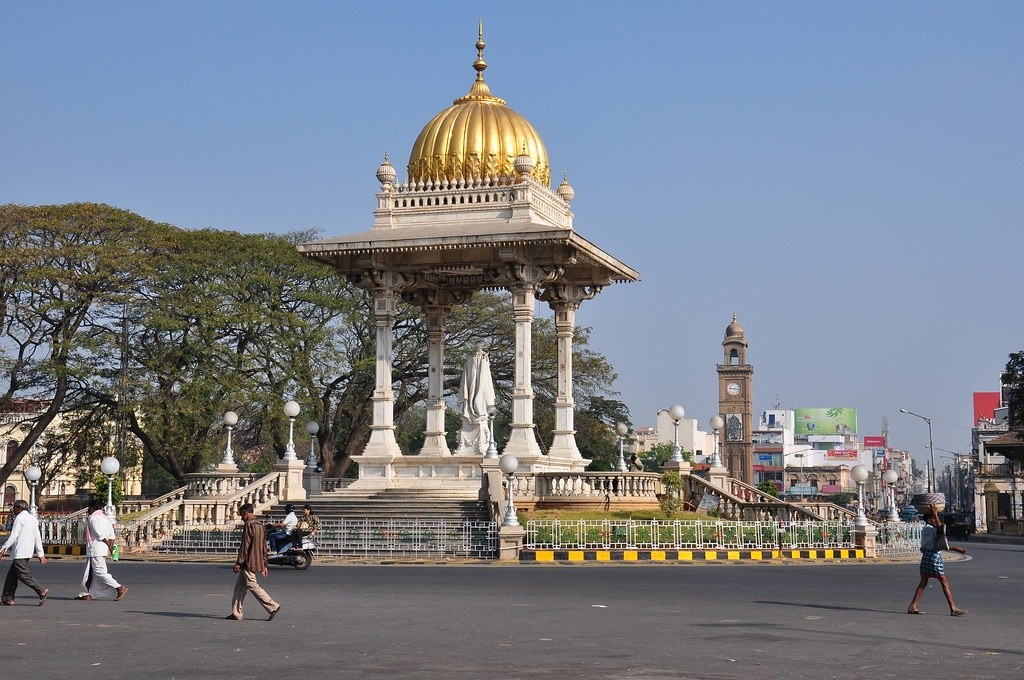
[[629, 453, 644, 473], [5, 502, 14, 531], [73, 498, 129, 601], [908, 503, 968, 616], [289, 505, 322, 551], [834, 538, 851, 547], [779, 519, 784, 526], [267, 504, 298, 555], [225, 503, 281, 621], [455, 343, 496, 425], [0, 500, 49, 606], [773, 513, 778, 521]]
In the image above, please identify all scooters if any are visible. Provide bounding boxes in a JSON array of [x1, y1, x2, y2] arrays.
[[263, 524, 321, 570]]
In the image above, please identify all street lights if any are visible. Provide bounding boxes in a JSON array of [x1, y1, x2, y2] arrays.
[[100, 457, 120, 516], [306, 421, 320, 469], [25, 466, 42, 519], [850, 464, 870, 526], [221, 411, 238, 464], [924, 445, 965, 513], [667, 404, 685, 462], [882, 469, 900, 522], [899, 408, 937, 494], [615, 423, 630, 472], [282, 401, 300, 460], [709, 416, 724, 467], [497, 454, 524, 528]]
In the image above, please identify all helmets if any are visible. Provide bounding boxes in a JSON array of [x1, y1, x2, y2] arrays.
[[284, 503, 294, 510]]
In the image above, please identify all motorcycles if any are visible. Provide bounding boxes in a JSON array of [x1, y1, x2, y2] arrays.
[[948, 513, 974, 541]]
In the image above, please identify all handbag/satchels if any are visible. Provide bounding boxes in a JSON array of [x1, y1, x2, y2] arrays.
[[298, 521, 308, 529], [936, 524, 949, 552]]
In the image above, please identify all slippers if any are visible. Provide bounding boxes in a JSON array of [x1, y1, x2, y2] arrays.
[[38, 590, 49, 606], [0, 602, 15, 606], [74, 595, 91, 600], [114, 587, 128, 601]]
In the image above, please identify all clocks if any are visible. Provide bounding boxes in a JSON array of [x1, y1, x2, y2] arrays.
[[727, 383, 741, 395]]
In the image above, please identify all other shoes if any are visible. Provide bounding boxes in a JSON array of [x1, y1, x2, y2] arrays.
[[268, 605, 281, 621], [289, 547, 303, 551], [951, 608, 968, 616], [267, 551, 277, 556], [226, 614, 235, 619], [908, 606, 925, 614]]
